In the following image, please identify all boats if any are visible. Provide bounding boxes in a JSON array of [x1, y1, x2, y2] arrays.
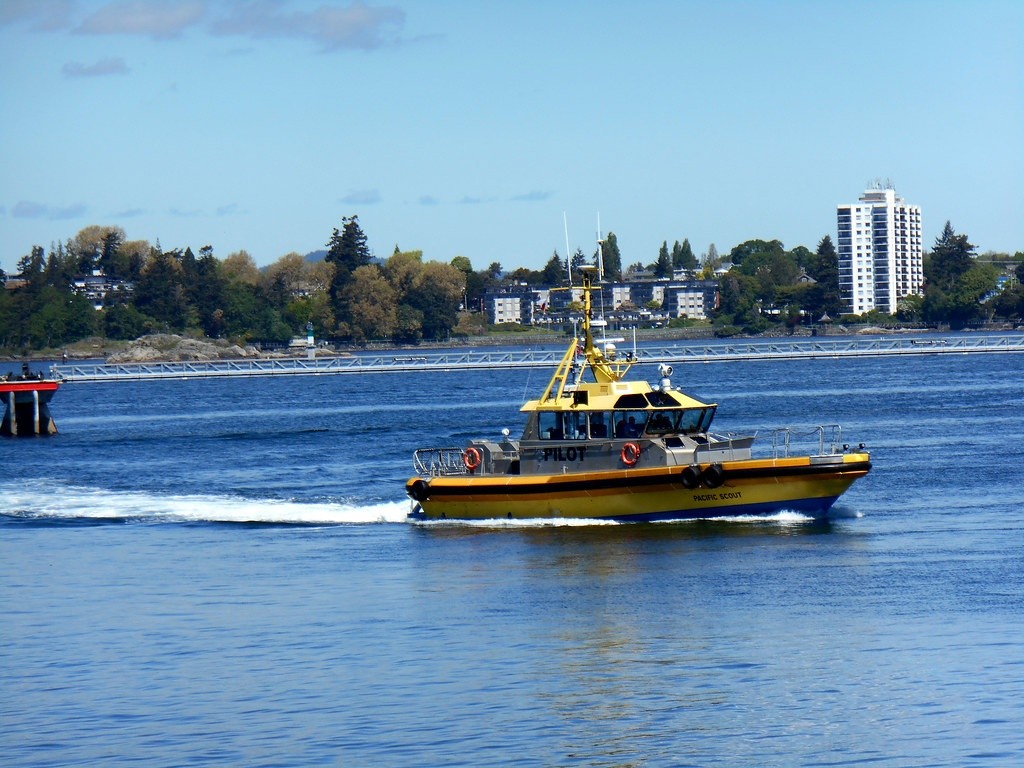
[[404, 208, 873, 522]]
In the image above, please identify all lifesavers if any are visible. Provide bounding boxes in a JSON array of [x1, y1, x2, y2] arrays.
[[680, 466, 702, 489], [410, 479, 430, 501], [702, 464, 724, 489], [620, 442, 640, 466], [463, 447, 481, 469]]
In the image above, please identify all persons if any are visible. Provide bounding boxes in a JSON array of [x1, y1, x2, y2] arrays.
[[619, 415, 669, 439]]
